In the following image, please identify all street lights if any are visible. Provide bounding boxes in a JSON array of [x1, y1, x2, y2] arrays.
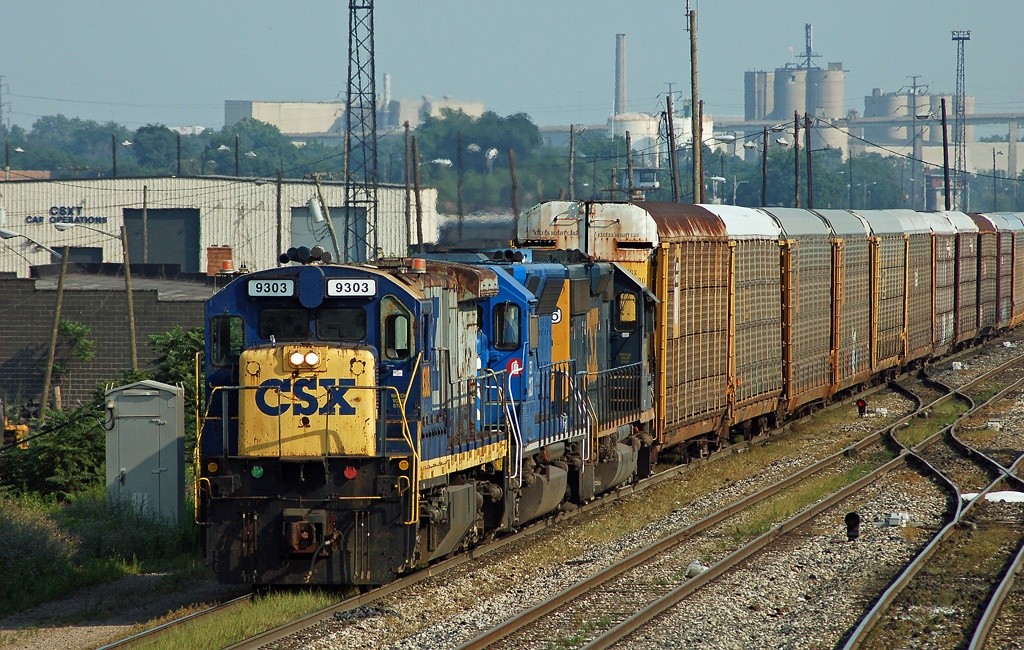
[[624, 129, 660, 206], [733, 175, 750, 206], [992, 147, 1004, 210], [411, 134, 452, 254], [217, 133, 256, 177], [5, 140, 25, 179], [847, 183, 861, 209], [863, 179, 877, 209], [112, 134, 133, 177], [699, 99, 736, 204], [743, 127, 789, 207]]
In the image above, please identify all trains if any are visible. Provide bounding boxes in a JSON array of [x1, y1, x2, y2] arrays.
[[191, 207, 1024, 589]]
